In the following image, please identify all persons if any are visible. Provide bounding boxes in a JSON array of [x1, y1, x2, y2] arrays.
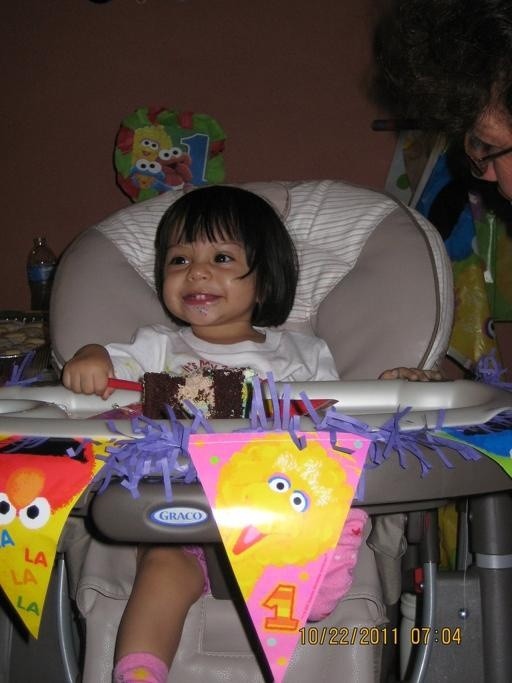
[[371, 0, 511, 391], [63, 180, 441, 680]]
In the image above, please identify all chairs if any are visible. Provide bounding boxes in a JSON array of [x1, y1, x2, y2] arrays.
[[1, 178, 511, 682]]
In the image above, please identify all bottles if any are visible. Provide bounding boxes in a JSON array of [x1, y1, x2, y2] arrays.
[[24, 235, 58, 311]]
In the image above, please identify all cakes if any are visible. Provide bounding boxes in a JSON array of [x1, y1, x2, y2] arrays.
[[141, 361, 258, 421]]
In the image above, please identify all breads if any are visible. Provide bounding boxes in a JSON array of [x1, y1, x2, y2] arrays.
[[0, 320, 45, 356]]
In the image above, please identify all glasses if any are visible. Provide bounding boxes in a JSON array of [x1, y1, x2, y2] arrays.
[[461, 129, 512, 174]]
